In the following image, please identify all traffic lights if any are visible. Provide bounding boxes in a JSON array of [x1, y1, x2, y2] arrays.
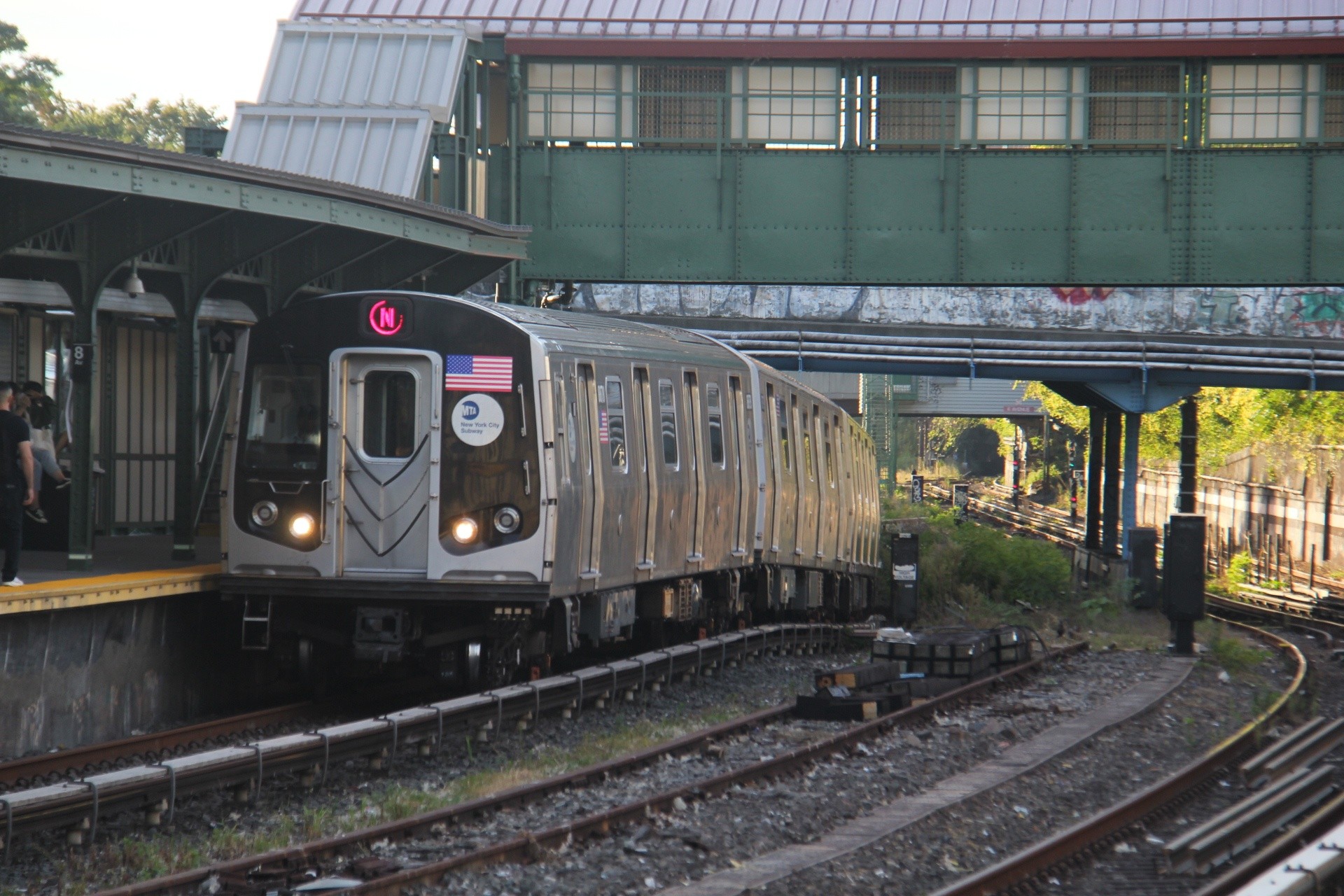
[[1012, 459, 1019, 471], [1012, 485, 1018, 493], [1069, 482, 1077, 503], [1068, 442, 1077, 468]]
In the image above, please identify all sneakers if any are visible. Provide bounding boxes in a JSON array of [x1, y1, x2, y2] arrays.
[[25, 507, 48, 525], [1, 576, 24, 588], [54, 477, 73, 490]]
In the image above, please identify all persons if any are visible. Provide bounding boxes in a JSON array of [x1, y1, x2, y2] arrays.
[[0, 380, 73, 586]]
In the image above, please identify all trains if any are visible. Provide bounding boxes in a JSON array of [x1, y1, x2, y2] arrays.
[[218, 287, 880, 684]]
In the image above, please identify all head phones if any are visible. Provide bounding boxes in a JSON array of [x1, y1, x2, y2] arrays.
[[122, 278, 144, 298]]
